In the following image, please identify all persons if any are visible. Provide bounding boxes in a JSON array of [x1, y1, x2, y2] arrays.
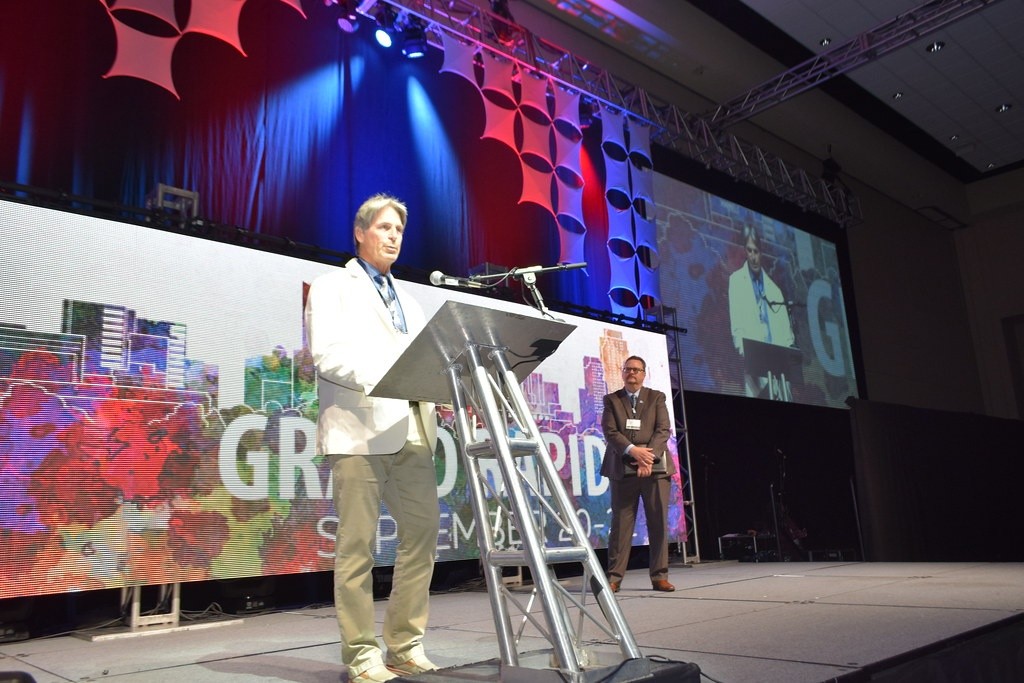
[[600, 355, 677, 592], [309, 192, 444, 683], [728, 222, 795, 402]]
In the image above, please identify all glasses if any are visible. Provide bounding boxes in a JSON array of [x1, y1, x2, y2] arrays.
[[621, 367, 645, 374]]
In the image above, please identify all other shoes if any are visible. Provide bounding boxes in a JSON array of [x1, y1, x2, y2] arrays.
[[348, 664, 398, 683], [386, 656, 442, 676]]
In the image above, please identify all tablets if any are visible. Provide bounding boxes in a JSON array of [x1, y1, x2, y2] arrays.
[[625, 444, 666, 474]]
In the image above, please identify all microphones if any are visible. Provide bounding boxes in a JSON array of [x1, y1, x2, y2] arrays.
[[429, 270, 487, 289], [760, 291, 774, 312]]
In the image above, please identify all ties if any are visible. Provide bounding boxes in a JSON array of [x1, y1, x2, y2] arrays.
[[629, 394, 637, 414], [754, 275, 767, 325], [375, 274, 406, 335]]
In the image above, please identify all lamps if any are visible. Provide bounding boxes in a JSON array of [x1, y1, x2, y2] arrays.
[[338, 0, 429, 58]]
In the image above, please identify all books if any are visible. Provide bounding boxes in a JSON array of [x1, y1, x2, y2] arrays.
[[623, 443, 667, 475]]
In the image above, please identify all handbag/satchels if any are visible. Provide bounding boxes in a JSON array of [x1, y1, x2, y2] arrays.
[[623, 443, 667, 476]]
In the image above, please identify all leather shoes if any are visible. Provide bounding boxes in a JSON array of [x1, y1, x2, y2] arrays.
[[651, 580, 675, 592], [609, 581, 621, 594]]
